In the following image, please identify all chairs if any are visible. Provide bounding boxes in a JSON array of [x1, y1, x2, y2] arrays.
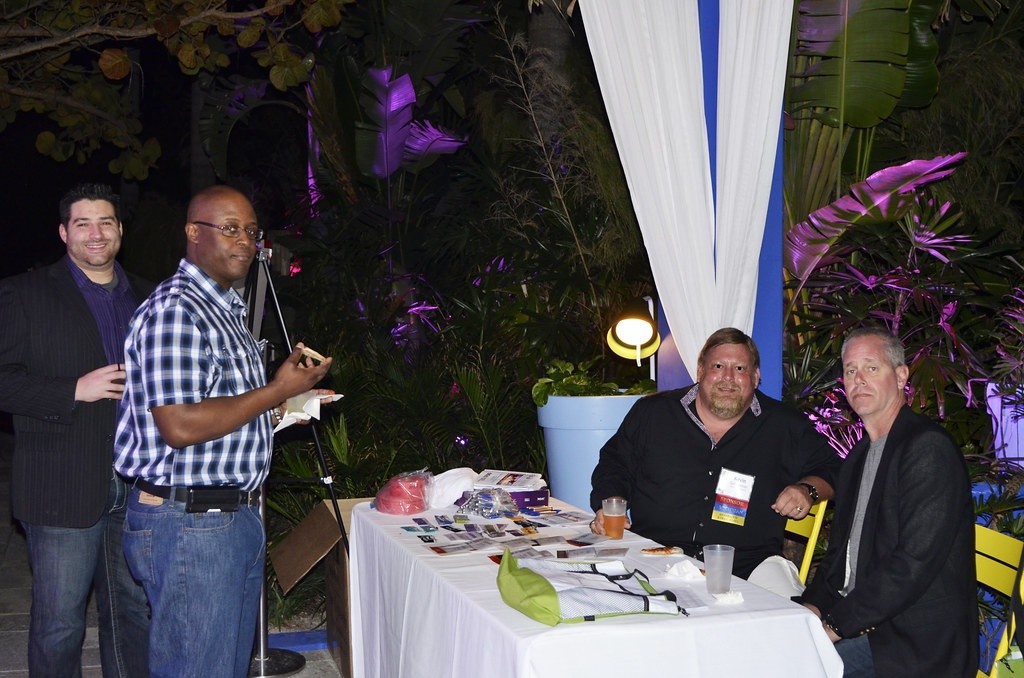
[[975, 524, 1024, 678], [785, 500, 827, 584]]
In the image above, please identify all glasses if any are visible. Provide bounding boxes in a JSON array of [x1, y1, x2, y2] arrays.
[[192, 220, 264, 241]]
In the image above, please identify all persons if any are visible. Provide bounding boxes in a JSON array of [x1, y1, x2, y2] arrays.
[[113, 183, 336, 678], [789, 324, 981, 678], [0, 183, 151, 678], [589, 327, 844, 581]]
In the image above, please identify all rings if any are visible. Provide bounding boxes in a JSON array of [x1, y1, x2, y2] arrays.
[[796, 506, 802, 513], [117, 363, 121, 370], [589, 520, 595, 528]]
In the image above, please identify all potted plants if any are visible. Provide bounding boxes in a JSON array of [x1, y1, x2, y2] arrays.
[[532, 354, 658, 515]]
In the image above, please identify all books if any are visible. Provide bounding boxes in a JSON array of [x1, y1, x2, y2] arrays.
[[473, 468, 548, 491]]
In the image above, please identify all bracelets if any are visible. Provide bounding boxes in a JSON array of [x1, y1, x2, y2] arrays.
[[273, 405, 282, 424], [825, 615, 843, 638]]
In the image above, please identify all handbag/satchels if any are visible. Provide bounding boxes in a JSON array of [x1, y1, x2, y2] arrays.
[[497, 549, 691, 626]]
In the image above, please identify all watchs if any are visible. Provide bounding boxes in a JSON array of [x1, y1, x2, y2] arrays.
[[799, 482, 818, 503]]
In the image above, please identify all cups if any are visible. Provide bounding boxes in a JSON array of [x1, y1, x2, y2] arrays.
[[704, 545, 735, 593], [601, 498, 627, 540]]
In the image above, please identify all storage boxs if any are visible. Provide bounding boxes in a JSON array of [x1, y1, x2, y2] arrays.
[[269, 497, 376, 678], [463, 489, 550, 508]]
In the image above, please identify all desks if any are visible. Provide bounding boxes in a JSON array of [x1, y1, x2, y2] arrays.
[[348, 498, 844, 678]]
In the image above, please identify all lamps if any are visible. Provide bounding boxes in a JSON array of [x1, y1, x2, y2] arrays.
[[606, 296, 661, 382]]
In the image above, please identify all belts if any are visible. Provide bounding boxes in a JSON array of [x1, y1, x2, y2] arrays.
[[125, 473, 262, 504]]
